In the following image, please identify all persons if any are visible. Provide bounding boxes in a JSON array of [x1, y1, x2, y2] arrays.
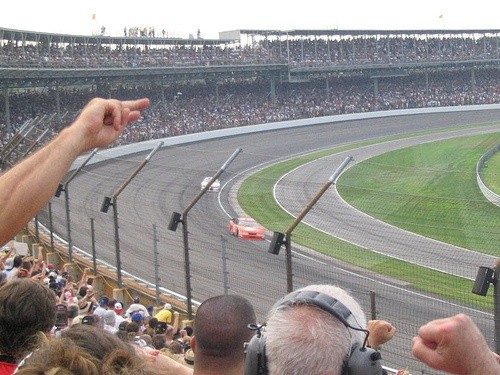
[[0, 97, 148, 250], [0, 280, 58, 375], [190, 294, 259, 375], [17, 324, 194, 375], [368, 320, 395, 349], [0, 27, 500, 172], [1, 245, 195, 369], [411, 314, 500, 375], [264, 284, 369, 375]]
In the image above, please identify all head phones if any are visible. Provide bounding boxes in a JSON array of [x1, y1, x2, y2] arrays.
[[243, 290, 382, 375]]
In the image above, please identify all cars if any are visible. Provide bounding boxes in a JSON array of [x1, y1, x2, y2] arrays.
[[201, 176, 221, 192], [229, 216, 267, 238]]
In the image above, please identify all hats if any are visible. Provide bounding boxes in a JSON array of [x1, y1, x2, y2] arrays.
[[180, 348, 195, 368], [114, 302, 124, 315], [82, 314, 98, 325], [131, 312, 145, 321], [48, 264, 55, 269], [118, 320, 129, 330], [100, 296, 108, 303], [147, 305, 154, 311], [164, 303, 172, 309], [55, 281, 64, 287], [5, 258, 14, 269], [134, 296, 139, 302], [49, 271, 58, 278], [156, 321, 168, 328]]
